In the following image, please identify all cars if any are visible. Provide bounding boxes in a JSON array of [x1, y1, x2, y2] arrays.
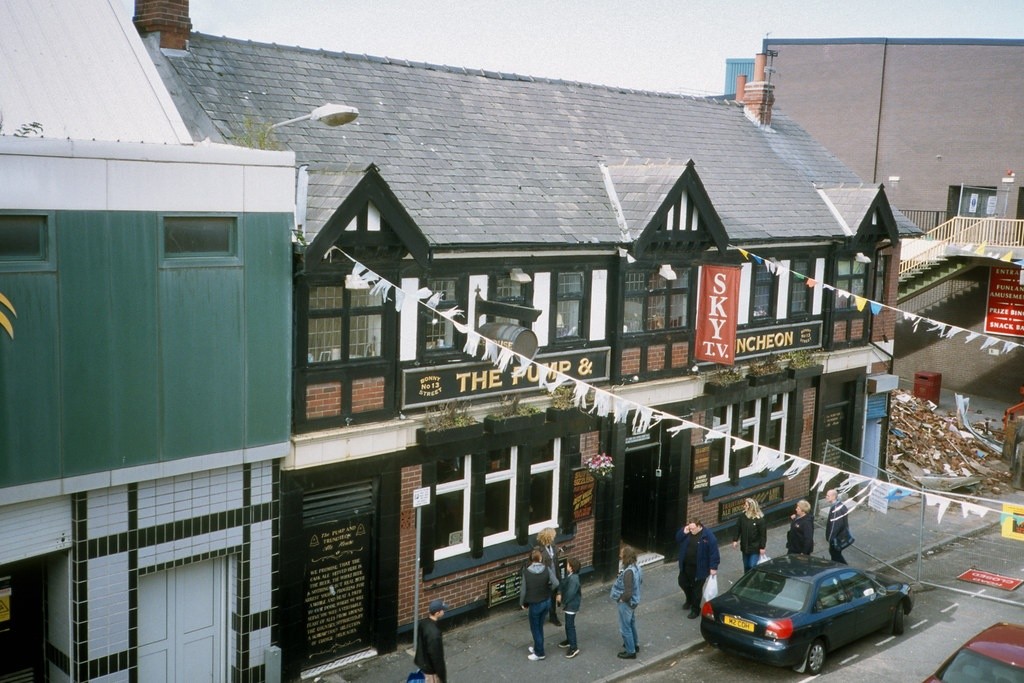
[[699, 552, 916, 677], [919, 621, 1024, 683]]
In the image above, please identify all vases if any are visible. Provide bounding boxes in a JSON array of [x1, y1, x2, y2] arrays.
[[594, 473, 612, 482]]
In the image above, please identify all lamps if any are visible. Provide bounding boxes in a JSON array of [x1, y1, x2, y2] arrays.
[[343, 274, 370, 290], [854, 252, 871, 264], [883, 334, 888, 343], [692, 365, 699, 375], [658, 264, 679, 281], [622, 375, 640, 385], [267, 101, 359, 127], [510, 267, 532, 283]]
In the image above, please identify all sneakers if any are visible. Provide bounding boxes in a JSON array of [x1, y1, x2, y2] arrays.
[[528, 647, 536, 654], [564, 646, 580, 658], [557, 639, 571, 648], [527, 654, 545, 660]]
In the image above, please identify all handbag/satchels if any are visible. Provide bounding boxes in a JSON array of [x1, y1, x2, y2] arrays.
[[699, 574, 718, 601], [403, 668, 426, 683], [831, 527, 855, 553], [756, 553, 772, 566]]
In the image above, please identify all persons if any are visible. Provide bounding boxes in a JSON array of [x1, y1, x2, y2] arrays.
[[785, 498, 816, 554], [825, 490, 855, 565], [520, 551, 559, 662], [610, 547, 639, 660], [413, 599, 447, 683], [733, 498, 767, 572], [558, 558, 583, 657], [532, 529, 564, 628], [675, 517, 720, 616]]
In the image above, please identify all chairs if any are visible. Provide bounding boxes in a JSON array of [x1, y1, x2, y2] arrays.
[[761, 573, 785, 595]]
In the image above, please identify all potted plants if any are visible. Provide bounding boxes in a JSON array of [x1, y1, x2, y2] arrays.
[[483, 391, 546, 434], [547, 386, 598, 423], [414, 397, 485, 448], [786, 347, 824, 378], [703, 364, 749, 396], [746, 352, 788, 388]]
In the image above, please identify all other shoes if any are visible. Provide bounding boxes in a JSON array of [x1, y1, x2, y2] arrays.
[[687, 607, 701, 619], [682, 601, 691, 610], [549, 618, 563, 626], [617, 651, 636, 659], [623, 642, 639, 652]]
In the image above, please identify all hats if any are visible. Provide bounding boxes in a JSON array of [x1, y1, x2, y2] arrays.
[[428, 600, 449, 612]]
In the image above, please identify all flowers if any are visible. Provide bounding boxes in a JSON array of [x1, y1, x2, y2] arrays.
[[583, 451, 616, 477]]
